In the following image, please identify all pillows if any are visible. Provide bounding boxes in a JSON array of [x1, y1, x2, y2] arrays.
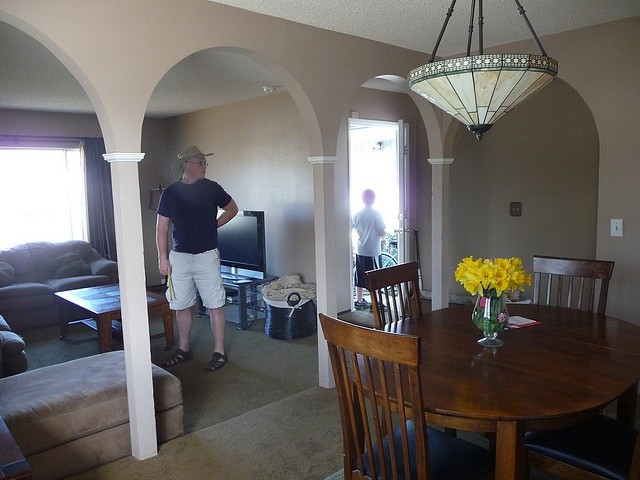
[[54, 249, 91, 278], [35, 252, 55, 279]]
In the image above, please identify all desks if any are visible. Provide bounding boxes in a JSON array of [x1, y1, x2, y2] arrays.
[[196, 274, 281, 330]]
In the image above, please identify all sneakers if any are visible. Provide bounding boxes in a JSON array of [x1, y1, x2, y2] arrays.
[[369, 300, 388, 312], [354, 298, 371, 310]]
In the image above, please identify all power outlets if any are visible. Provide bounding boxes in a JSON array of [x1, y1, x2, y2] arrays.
[[609, 216, 623, 236], [509, 200, 522, 216]]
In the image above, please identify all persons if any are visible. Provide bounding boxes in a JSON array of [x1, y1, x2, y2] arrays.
[[155, 145, 238, 372], [353, 189, 388, 312]]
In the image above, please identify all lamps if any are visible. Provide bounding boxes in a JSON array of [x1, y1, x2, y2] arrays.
[[147, 184, 166, 212], [408, 0, 559, 140]]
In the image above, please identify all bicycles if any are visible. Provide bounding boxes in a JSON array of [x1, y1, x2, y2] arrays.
[[381, 240, 398, 290]]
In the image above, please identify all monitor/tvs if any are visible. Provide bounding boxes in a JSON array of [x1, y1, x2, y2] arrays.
[[216, 210, 265, 280]]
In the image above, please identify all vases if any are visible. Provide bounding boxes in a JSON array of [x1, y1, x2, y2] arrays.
[[471, 294, 510, 349]]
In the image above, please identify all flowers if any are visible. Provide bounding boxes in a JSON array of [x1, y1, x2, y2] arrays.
[[454, 253, 533, 336]]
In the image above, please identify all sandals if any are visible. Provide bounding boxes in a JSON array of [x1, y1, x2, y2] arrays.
[[205, 351, 227, 370], [161, 346, 192, 367]]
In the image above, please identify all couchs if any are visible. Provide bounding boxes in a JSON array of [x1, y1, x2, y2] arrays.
[[0, 240, 119, 377], [0, 351, 185, 478]]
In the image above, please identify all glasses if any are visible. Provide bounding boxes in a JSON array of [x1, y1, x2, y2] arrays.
[[188, 160, 209, 167]]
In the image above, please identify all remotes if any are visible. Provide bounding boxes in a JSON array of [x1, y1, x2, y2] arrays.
[[233, 280, 254, 284]]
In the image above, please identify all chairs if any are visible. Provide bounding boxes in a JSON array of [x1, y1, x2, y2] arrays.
[[523, 411, 638, 480], [363, 261, 456, 439], [317, 314, 495, 480], [534, 255, 615, 316]]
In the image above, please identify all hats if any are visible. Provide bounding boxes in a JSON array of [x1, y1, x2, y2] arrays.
[[170, 145, 214, 175]]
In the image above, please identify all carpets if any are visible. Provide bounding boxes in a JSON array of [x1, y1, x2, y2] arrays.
[[324, 420, 640, 479]]
